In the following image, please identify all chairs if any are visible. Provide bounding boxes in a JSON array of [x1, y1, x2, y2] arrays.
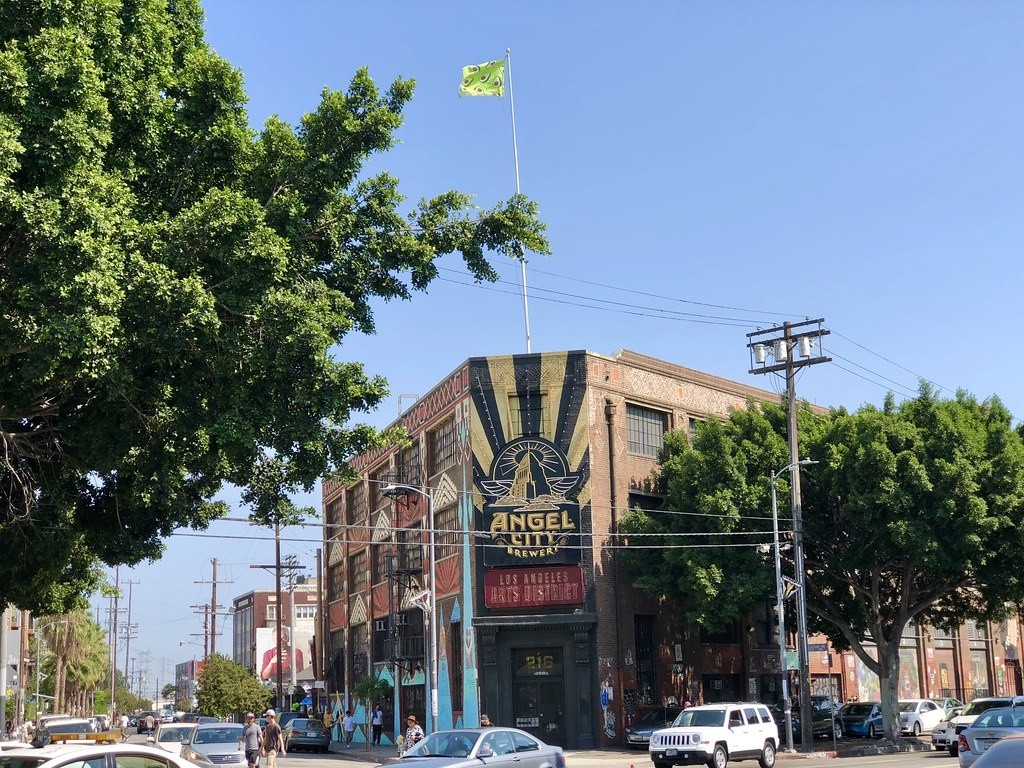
[[444, 737, 468, 756], [489, 739, 501, 755]]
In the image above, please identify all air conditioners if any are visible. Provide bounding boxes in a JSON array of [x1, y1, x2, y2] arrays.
[[394, 615, 407, 626], [376, 621, 388, 631]]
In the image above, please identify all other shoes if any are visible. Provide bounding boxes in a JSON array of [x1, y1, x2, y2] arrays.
[[347, 744, 350, 749], [373, 741, 375, 746], [377, 743, 381, 747]]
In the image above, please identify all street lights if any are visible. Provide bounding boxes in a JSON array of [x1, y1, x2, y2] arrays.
[[770, 458, 822, 750], [379, 436, 424, 745], [35, 620, 67, 740], [180, 640, 215, 671]]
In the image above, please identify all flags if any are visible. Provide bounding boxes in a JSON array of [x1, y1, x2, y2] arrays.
[[458, 59, 505, 97]]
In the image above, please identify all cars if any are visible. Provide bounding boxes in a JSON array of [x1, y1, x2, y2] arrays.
[[371, 727, 567, 768], [958, 705, 1024, 768], [126, 716, 138, 727], [833, 700, 883, 739], [925, 697, 966, 718], [898, 699, 945, 735], [180, 723, 261, 768], [279, 717, 333, 754], [275, 712, 310, 730], [0, 715, 201, 768], [143, 722, 200, 758], [254, 719, 270, 731], [931, 706, 968, 751], [136, 711, 160, 734], [162, 713, 221, 725], [625, 703, 687, 749], [967, 737, 1024, 768], [769, 694, 846, 741], [945, 696, 1024, 758]]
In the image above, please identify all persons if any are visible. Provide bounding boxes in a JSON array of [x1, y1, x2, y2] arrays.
[[371, 704, 383, 747], [323, 708, 357, 748], [402, 716, 429, 754], [101, 716, 111, 732], [118, 712, 129, 742], [238, 709, 287, 768], [6, 719, 11, 733], [145, 713, 154, 737], [24, 721, 36, 742], [481, 714, 494, 739], [260, 626, 302, 682], [684, 702, 692, 709]]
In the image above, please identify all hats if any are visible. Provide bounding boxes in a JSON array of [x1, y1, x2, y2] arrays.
[[684, 701, 692, 708], [404, 715, 418, 724], [481, 714, 489, 722], [246, 713, 254, 717], [263, 709, 276, 717]]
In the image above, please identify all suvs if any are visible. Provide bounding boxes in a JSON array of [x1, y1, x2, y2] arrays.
[[648, 702, 780, 768]]
